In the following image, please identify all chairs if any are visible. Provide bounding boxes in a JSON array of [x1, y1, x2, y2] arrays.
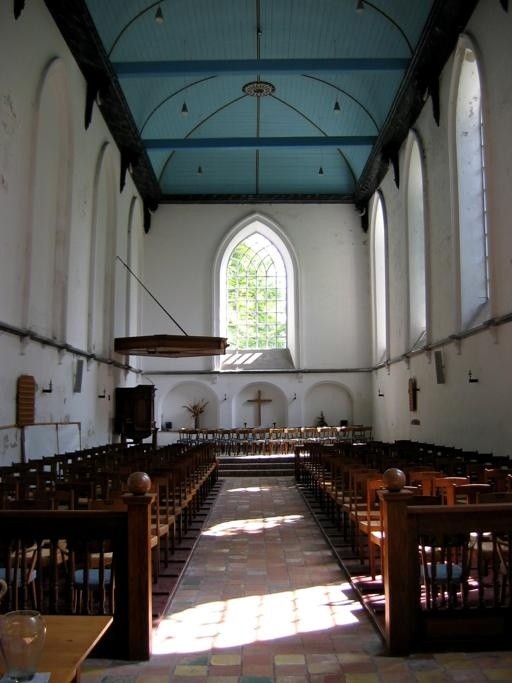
[[0, 439, 221, 616], [176, 427, 374, 455], [292, 438, 510, 613]]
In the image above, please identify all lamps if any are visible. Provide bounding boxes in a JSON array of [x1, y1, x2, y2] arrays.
[[154, 0, 366, 174]]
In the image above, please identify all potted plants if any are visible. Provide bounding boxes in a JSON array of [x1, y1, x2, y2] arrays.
[[181, 399, 209, 428]]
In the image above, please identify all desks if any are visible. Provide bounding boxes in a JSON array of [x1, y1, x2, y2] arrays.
[[0, 615, 113, 683]]
